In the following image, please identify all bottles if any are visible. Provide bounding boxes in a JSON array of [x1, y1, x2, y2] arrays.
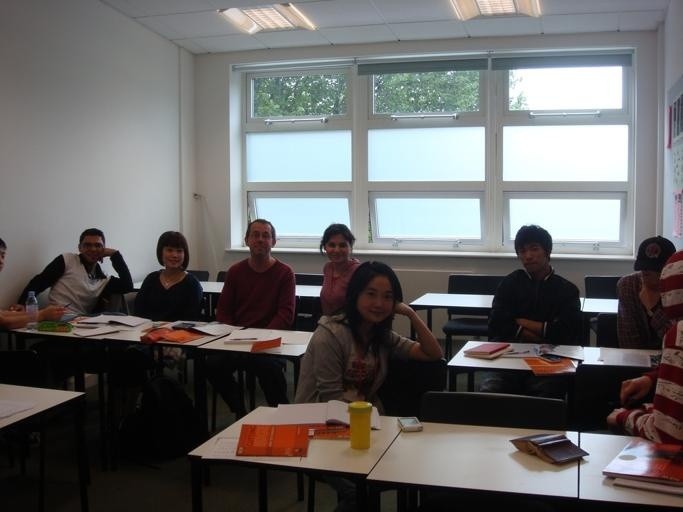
[[24, 291, 38, 329]]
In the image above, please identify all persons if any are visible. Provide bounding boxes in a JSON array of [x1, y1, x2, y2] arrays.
[[0, 238, 66, 330], [9, 228, 133, 368], [293, 260, 443, 511], [477, 225, 586, 424], [208, 219, 297, 421], [609, 252, 682, 448], [320, 222, 358, 313], [131, 230, 203, 409]]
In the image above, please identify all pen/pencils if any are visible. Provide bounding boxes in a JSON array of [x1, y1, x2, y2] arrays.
[[228, 338, 257, 341], [62, 302, 73, 309]]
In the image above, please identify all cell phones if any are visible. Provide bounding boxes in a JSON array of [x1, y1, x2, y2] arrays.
[[396, 416, 423, 432], [172, 323, 195, 330]]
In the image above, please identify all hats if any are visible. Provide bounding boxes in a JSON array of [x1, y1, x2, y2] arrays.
[[634, 235, 675, 272]]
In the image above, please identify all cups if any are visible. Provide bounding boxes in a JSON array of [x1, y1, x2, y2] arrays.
[[346, 401, 373, 450]]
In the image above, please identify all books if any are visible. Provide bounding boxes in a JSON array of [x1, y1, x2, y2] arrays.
[[507, 432, 682, 496], [463, 341, 579, 380], [232, 399, 382, 459], [71, 312, 308, 353]]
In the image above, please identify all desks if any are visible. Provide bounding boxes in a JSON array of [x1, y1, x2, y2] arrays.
[[576, 430, 682, 512], [181, 403, 406, 512], [0, 382, 94, 512], [364, 421, 578, 512]]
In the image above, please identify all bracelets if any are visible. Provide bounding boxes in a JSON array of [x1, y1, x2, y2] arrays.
[[645, 304, 657, 316], [615, 237, 676, 405]]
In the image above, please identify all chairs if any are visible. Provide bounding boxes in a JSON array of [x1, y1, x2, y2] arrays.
[[414, 386, 569, 512], [301, 360, 448, 512]]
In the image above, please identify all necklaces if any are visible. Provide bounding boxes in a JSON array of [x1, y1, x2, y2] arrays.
[[161, 272, 177, 288]]
